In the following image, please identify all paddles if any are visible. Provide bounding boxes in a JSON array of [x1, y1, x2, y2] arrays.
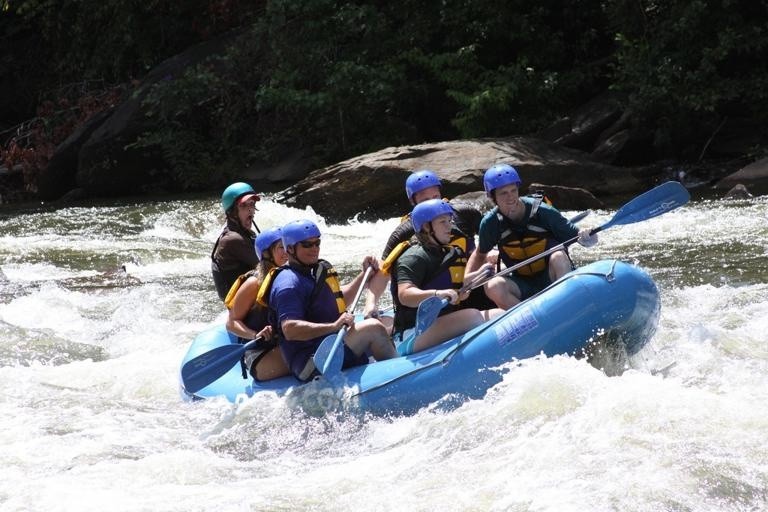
[[181, 336, 266, 393], [415, 269, 489, 339], [312, 265, 375, 385], [567, 210, 590, 224], [466, 182, 691, 292]]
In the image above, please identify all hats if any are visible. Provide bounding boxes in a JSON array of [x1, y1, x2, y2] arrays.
[[236, 193, 259, 204]]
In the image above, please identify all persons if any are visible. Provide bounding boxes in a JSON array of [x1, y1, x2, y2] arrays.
[[362, 169, 484, 319], [211, 182, 261, 303], [392, 198, 504, 355], [463, 163, 600, 310], [225, 224, 294, 382], [266, 220, 398, 384]]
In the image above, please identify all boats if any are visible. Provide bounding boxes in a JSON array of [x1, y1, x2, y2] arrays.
[[177, 254, 664, 425]]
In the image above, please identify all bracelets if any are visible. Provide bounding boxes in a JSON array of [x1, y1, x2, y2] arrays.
[[254, 333, 258, 340], [435, 290, 437, 296]]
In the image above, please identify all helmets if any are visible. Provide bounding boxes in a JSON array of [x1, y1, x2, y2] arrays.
[[222, 182, 255, 214], [411, 198, 452, 234], [405, 170, 440, 206], [254, 226, 282, 261], [281, 219, 319, 249], [483, 163, 520, 192]]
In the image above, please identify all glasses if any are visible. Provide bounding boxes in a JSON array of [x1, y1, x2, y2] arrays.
[[299, 239, 319, 248]]
